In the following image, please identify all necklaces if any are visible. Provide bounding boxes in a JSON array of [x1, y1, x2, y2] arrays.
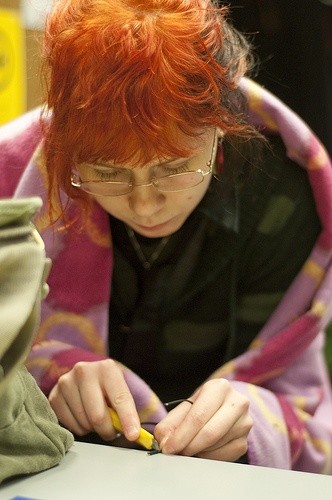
[[127, 227, 168, 269]]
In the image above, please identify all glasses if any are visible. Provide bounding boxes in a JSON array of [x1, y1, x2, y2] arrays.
[[70, 127, 219, 199]]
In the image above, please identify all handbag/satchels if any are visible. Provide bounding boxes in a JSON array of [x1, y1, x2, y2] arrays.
[[0, 197, 74, 483]]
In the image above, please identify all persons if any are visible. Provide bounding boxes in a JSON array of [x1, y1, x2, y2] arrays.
[[0, 0, 332, 475]]
